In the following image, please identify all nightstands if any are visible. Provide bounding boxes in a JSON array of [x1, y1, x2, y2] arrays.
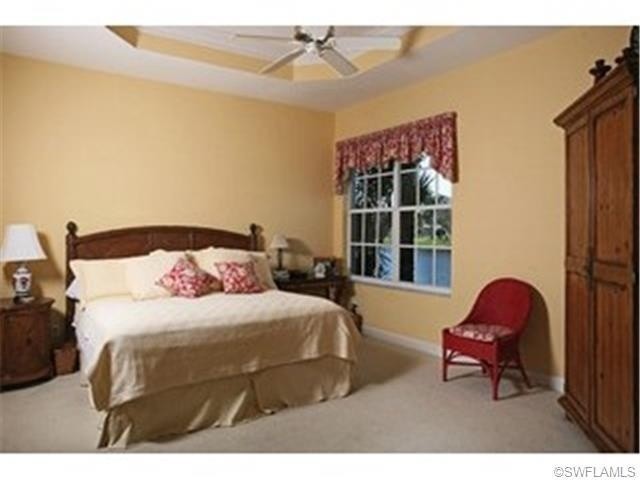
[[271, 270, 346, 301], [0, 297, 55, 390]]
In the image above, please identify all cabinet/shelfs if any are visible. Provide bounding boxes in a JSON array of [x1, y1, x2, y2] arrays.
[[549, 26, 640, 453]]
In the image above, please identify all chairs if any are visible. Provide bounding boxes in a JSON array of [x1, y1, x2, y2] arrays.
[[440, 277, 535, 400]]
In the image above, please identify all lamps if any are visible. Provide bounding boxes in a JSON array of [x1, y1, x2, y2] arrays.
[[269, 235, 290, 269], [0, 222, 49, 304]]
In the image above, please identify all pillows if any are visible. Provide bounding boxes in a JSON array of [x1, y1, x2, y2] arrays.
[[68, 246, 276, 300]]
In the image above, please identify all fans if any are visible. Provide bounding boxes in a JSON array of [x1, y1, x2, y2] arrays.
[[235, 25, 402, 77]]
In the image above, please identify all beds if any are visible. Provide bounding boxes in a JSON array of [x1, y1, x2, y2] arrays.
[[62, 220, 362, 450]]
